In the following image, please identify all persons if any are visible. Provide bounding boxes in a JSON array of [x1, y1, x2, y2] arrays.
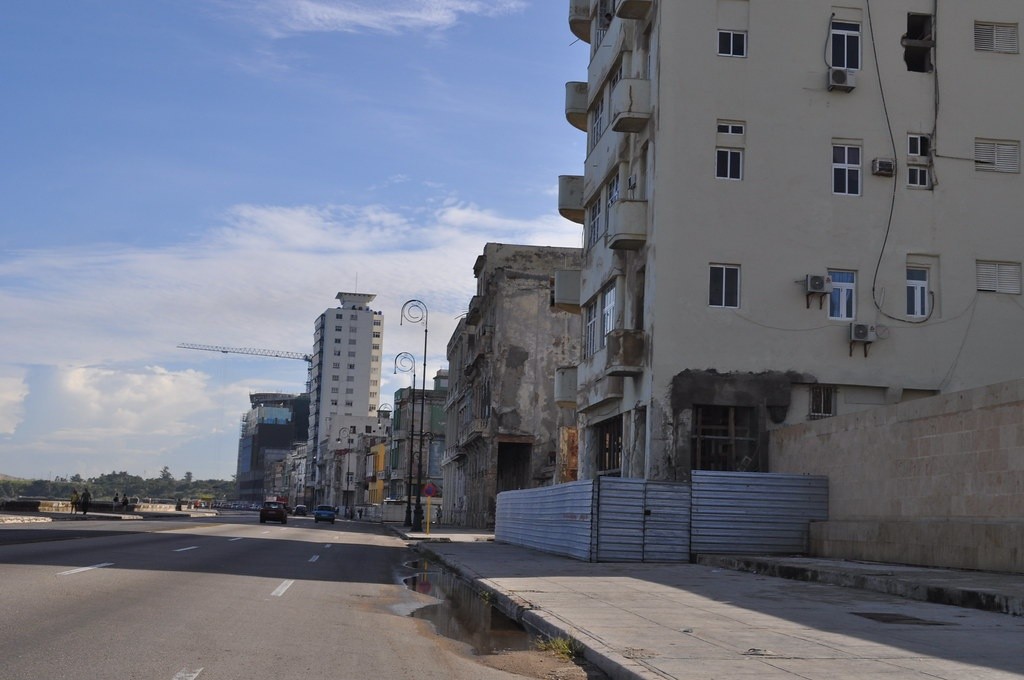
[[177, 499, 182, 511], [81, 488, 91, 515], [113, 493, 119, 513], [346, 505, 354, 520], [121, 493, 128, 513], [69, 489, 79, 515]]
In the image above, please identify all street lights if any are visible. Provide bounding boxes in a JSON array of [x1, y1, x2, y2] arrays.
[[335, 427, 351, 519], [400, 300, 428, 532], [393, 353, 412, 528], [377, 404, 394, 500]]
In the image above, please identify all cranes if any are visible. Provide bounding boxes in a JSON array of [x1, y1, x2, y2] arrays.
[[177, 342, 313, 393]]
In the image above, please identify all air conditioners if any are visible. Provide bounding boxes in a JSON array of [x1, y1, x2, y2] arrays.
[[875, 158, 893, 175], [851, 321, 877, 344], [828, 68, 857, 88], [806, 273, 833, 293]]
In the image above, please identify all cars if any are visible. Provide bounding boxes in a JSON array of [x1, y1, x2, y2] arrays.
[[260, 501, 287, 524], [200, 502, 205, 508], [314, 505, 336, 525], [292, 505, 307, 517], [212, 502, 263, 511], [286, 507, 294, 514]]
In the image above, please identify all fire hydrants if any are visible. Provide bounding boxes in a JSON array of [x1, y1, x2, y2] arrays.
[[357, 508, 364, 520]]
